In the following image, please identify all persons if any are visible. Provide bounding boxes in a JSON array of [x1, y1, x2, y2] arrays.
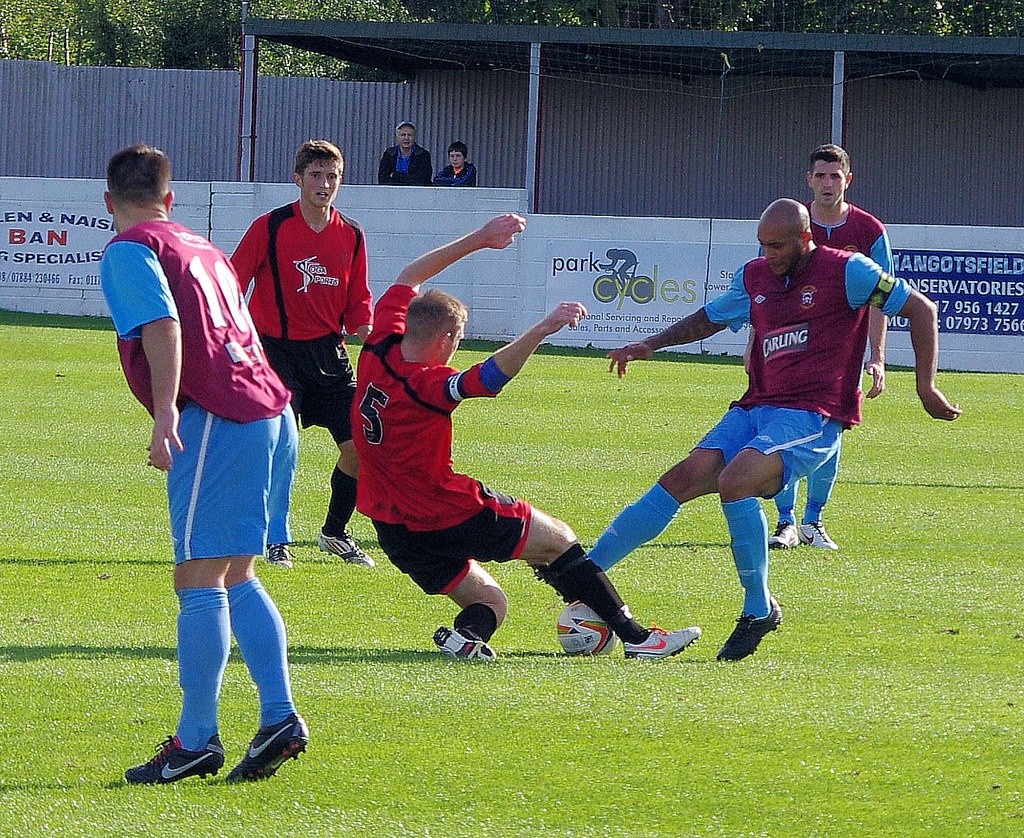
[[377, 120, 433, 184], [589, 198, 963, 662], [433, 141, 476, 188], [98, 144, 309, 786], [230, 140, 375, 569], [351, 214, 702, 660], [744, 144, 895, 550]]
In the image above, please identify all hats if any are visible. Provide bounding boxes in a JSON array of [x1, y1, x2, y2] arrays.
[[396, 120, 416, 132]]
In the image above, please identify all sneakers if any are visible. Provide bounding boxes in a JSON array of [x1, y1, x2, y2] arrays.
[[433, 626, 497, 659], [717, 596, 783, 661], [624, 622, 701, 660], [319, 527, 374, 566], [800, 517, 837, 550], [268, 543, 296, 569], [125, 733, 225, 784], [768, 520, 800, 550], [529, 562, 578, 606], [226, 713, 310, 783]]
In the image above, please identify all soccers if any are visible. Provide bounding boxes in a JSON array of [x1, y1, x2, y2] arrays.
[[556, 596, 620, 657]]
